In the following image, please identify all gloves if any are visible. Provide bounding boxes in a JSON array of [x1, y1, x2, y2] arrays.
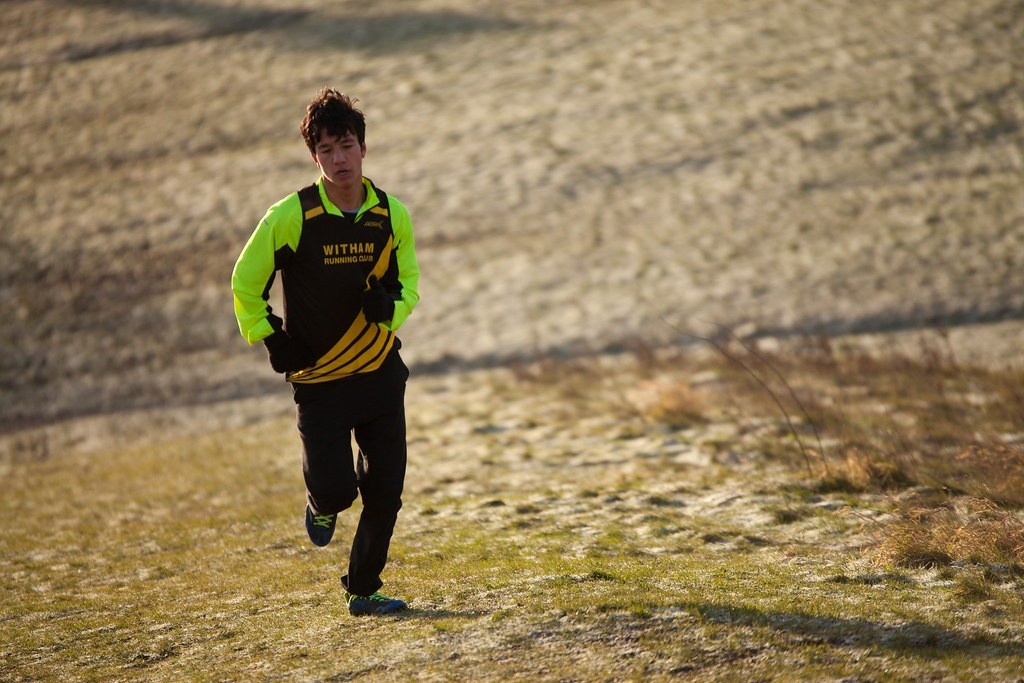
[[359, 273, 405, 327], [264, 331, 318, 374]]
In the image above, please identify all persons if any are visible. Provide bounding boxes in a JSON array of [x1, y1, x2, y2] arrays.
[[232, 87, 420, 615]]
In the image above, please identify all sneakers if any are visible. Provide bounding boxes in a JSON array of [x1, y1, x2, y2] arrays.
[[305, 505, 345, 548], [343, 592, 406, 615]]
